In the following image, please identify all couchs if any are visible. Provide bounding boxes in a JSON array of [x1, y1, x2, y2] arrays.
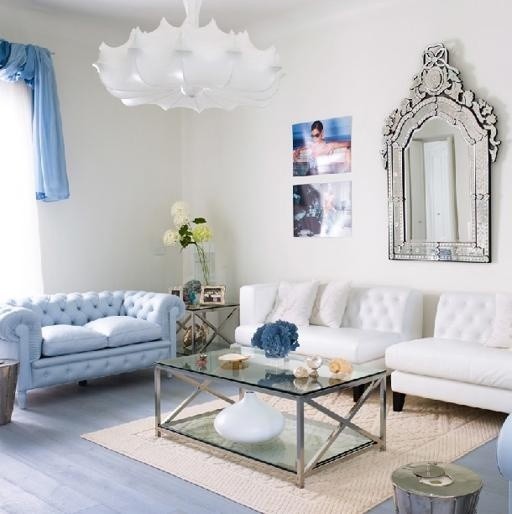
[[234, 283, 512, 416], [495, 416, 512, 513], [2, 287, 186, 411]]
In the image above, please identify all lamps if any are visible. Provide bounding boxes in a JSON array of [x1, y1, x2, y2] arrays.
[[91, 0, 282, 115]]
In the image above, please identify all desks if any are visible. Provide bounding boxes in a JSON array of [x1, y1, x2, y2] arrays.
[[177, 301, 239, 356]]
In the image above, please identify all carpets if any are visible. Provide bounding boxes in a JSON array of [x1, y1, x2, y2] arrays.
[[80, 375, 509, 514]]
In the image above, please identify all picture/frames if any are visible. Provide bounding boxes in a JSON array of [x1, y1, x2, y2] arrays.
[[200, 285, 226, 305], [169, 287, 183, 298]]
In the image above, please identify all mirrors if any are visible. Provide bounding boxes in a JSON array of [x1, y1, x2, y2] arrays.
[[380, 42, 500, 263]]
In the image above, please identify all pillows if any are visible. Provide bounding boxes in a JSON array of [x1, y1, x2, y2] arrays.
[[263, 280, 349, 327]]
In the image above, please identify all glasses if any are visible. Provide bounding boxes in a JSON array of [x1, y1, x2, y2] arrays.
[[311, 133, 322, 137]]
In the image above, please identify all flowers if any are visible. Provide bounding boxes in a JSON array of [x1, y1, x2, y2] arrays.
[[161, 203, 217, 285]]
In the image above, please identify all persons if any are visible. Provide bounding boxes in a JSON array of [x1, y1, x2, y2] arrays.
[[293, 120, 349, 176]]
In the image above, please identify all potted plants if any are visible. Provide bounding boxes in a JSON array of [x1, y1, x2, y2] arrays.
[[252, 321, 300, 376]]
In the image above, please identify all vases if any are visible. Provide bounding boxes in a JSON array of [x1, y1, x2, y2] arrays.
[[189, 248, 218, 287]]
